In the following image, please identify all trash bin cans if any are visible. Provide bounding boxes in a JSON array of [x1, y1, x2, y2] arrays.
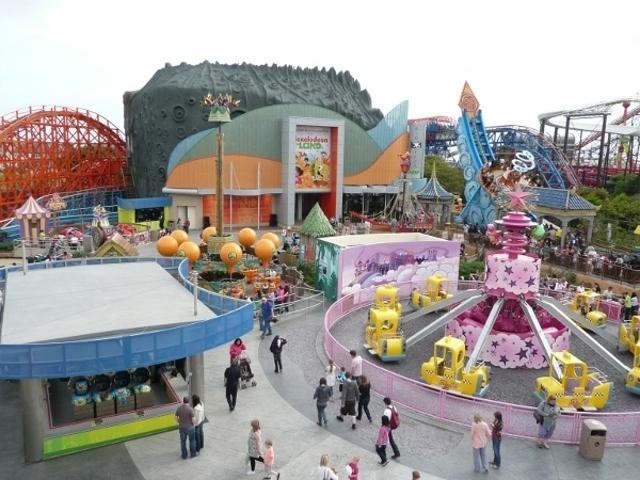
[[579, 419, 608, 461]]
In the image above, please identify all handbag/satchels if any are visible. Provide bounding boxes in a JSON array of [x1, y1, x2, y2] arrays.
[[533, 410, 543, 425]]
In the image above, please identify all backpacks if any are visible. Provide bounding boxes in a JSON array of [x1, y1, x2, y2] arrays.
[[387, 406, 400, 429]]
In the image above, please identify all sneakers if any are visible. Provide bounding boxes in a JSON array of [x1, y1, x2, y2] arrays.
[[537, 439, 549, 449], [317, 416, 372, 430], [246, 470, 281, 480], [380, 453, 400, 467], [474, 462, 500, 473]]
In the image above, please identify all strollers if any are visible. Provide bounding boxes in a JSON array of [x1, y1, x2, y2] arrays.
[[231, 355, 257, 389]]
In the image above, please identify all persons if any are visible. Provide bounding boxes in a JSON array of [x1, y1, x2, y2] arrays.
[[319, 454, 338, 480], [374, 416, 391, 466], [312, 349, 374, 429], [262, 440, 281, 480], [412, 470, 422, 480], [256, 282, 290, 340], [537, 394, 562, 449], [382, 397, 400, 459], [191, 394, 206, 456], [175, 396, 199, 460], [270, 334, 288, 373], [345, 456, 362, 480], [223, 358, 242, 411], [228, 337, 247, 366], [246, 419, 264, 475], [535, 222, 640, 320], [390, 216, 399, 233], [470, 413, 493, 473], [487, 410, 505, 469]]
